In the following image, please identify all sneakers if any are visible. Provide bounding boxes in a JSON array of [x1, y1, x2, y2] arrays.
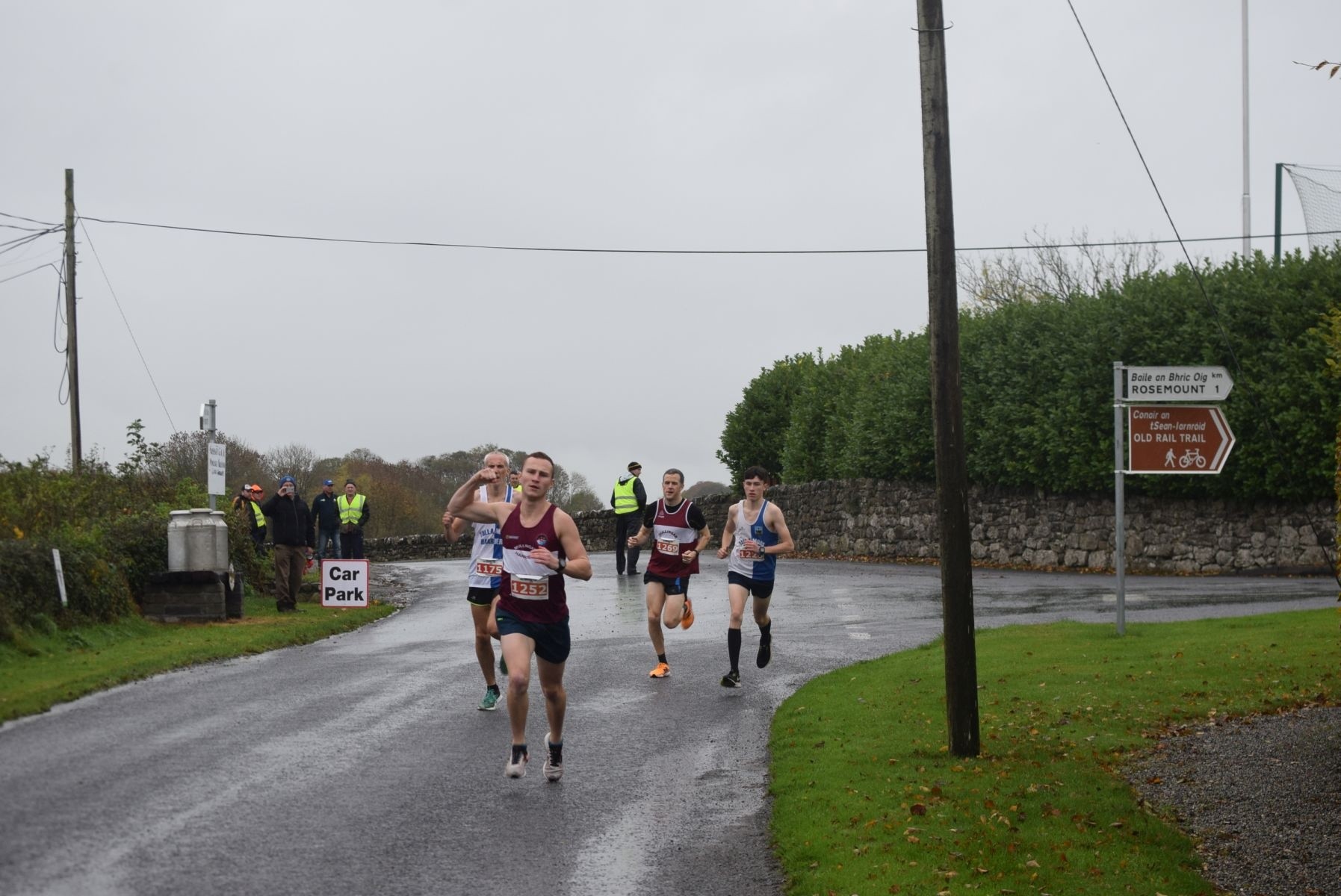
[[720, 671, 741, 687], [477, 687, 502, 710], [499, 653, 508, 675], [757, 634, 771, 668], [650, 663, 671, 678], [543, 731, 564, 781], [680, 599, 694, 629], [503, 743, 529, 779]]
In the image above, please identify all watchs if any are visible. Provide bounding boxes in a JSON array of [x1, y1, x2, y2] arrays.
[[758, 544, 765, 556], [693, 550, 699, 558], [555, 558, 566, 573]]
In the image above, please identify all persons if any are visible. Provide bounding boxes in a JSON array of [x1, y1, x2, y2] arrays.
[[232, 483, 269, 559], [718, 467, 794, 687], [628, 468, 710, 678], [312, 480, 342, 568], [261, 474, 315, 614], [335, 478, 370, 559], [610, 462, 647, 575], [442, 451, 592, 781]]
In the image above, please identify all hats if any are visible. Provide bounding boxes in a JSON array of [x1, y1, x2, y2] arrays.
[[628, 462, 642, 472], [279, 476, 296, 489], [322, 479, 334, 485], [241, 485, 254, 491], [345, 479, 356, 487], [251, 484, 263, 492]]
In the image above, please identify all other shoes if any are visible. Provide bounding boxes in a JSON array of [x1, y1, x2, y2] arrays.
[[617, 570, 623, 574], [627, 572, 640, 575], [292, 608, 306, 614]]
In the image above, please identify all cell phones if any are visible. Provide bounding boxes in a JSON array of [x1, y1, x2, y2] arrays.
[[286, 487, 294, 493]]
